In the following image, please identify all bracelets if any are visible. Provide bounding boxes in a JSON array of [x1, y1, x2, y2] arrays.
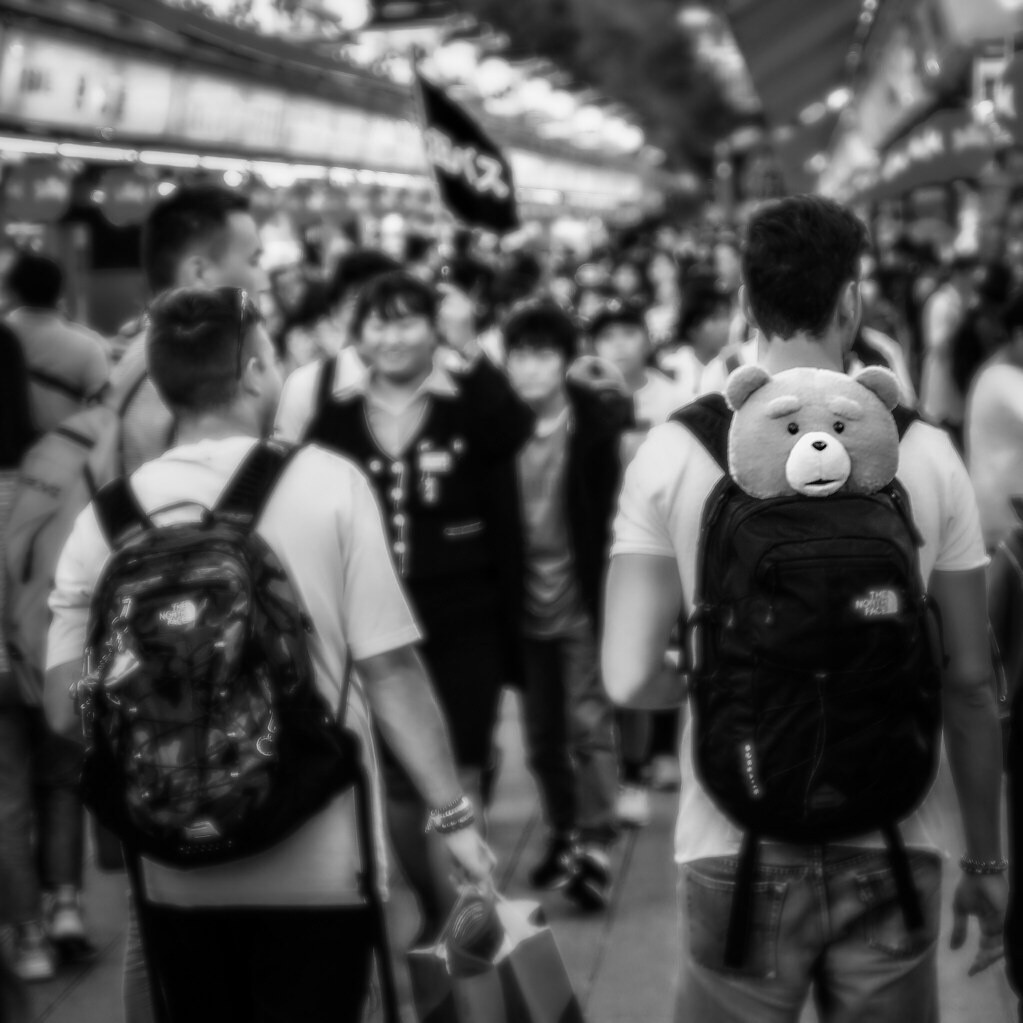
[[429, 795, 475, 833], [958, 855, 1008, 877]]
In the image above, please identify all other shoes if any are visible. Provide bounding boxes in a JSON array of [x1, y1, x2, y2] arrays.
[[570, 847, 619, 913], [52, 909, 88, 964], [529, 836, 576, 889], [0, 924, 54, 979]]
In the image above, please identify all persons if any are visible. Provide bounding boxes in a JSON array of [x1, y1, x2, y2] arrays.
[[0, 183, 1023, 1023]]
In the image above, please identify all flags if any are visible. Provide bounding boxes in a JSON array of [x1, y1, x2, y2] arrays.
[[418, 73, 522, 235]]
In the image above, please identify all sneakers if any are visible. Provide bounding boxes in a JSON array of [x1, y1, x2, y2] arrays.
[[616, 785, 652, 829], [655, 756, 681, 789]]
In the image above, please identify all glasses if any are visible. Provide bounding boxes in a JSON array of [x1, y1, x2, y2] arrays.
[[219, 286, 259, 395]]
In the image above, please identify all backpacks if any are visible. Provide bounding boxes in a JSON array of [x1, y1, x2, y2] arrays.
[[75, 440, 362, 872], [668, 394, 948, 845]]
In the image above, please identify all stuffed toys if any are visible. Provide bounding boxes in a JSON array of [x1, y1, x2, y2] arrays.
[[723, 365, 900, 500]]
